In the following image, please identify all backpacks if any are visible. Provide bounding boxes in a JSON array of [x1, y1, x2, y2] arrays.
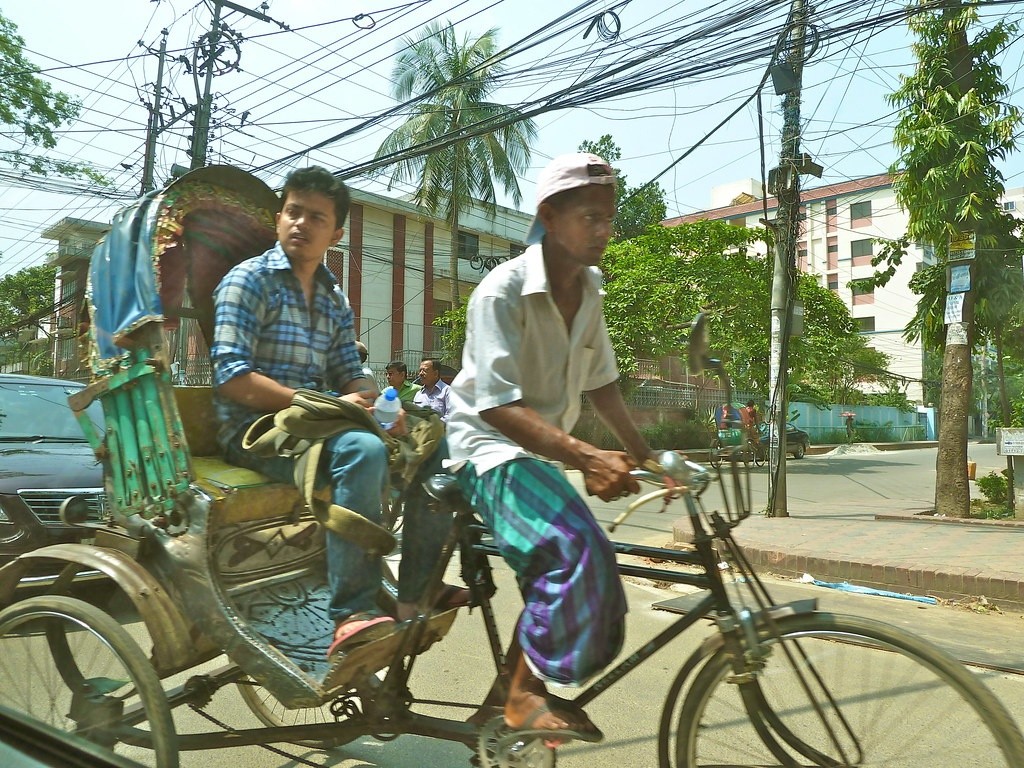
[[241, 388, 446, 555]]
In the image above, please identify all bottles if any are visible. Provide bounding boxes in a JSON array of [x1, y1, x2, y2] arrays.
[[373, 389, 402, 431]]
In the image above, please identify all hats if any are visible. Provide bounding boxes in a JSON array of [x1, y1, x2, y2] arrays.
[[524, 153, 617, 245]]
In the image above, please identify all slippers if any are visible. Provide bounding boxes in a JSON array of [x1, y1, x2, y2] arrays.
[[429, 585, 497, 617], [497, 700, 602, 750], [326, 613, 397, 658]]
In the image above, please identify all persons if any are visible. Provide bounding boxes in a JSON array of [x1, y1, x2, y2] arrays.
[[381, 361, 422, 403], [210, 165, 498, 658], [441, 153, 688, 759], [413, 358, 451, 423], [745, 399, 758, 443], [355, 341, 380, 404]]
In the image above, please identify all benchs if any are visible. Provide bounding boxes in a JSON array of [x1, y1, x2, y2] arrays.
[[164, 383, 308, 524]]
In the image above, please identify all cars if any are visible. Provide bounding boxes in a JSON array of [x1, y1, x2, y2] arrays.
[[0, 372, 112, 588], [738, 421, 811, 463]]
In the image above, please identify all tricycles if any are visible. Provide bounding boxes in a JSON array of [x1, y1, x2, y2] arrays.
[[708, 401, 769, 469], [0, 162, 1024, 767]]
